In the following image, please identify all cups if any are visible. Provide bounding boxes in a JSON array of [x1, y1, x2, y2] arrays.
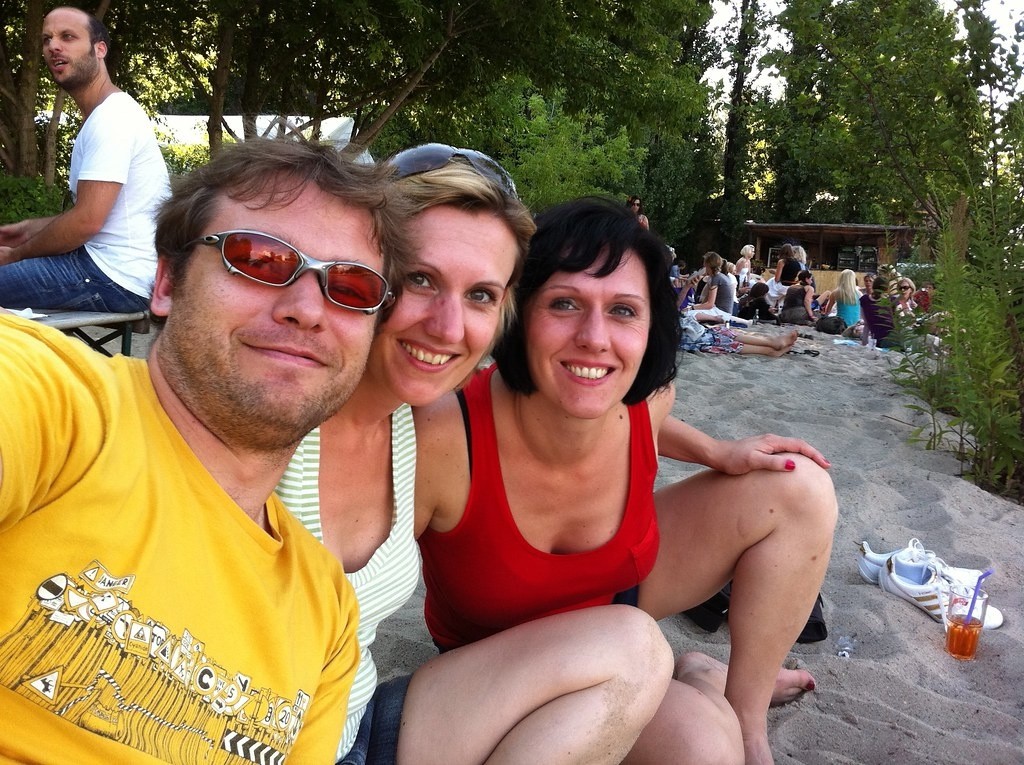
[[868, 338, 878, 351], [946, 585, 989, 660]]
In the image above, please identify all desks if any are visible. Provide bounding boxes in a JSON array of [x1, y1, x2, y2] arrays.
[[761, 268, 867, 296]]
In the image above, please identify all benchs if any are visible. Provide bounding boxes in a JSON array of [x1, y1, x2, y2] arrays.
[[15, 309, 150, 356]]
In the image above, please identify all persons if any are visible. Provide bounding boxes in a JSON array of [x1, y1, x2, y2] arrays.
[[0, 6, 174, 315], [0, 131, 359, 765], [667, 244, 952, 358], [825, 269, 864, 327], [624, 196, 649, 231], [781, 269, 821, 324], [414, 196, 837, 764], [280, 144, 675, 763]]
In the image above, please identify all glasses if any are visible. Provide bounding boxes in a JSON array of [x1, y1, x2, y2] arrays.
[[179, 229, 395, 315], [898, 286, 911, 291], [632, 203, 640, 206], [374, 142, 516, 197]]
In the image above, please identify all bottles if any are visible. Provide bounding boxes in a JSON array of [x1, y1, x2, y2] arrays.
[[753, 309, 760, 325]]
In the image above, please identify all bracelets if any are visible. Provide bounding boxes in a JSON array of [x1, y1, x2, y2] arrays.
[[810, 315, 815, 318]]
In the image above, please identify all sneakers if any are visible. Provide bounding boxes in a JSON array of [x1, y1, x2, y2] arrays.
[[857, 538, 985, 588], [879, 553, 1003, 630]]
